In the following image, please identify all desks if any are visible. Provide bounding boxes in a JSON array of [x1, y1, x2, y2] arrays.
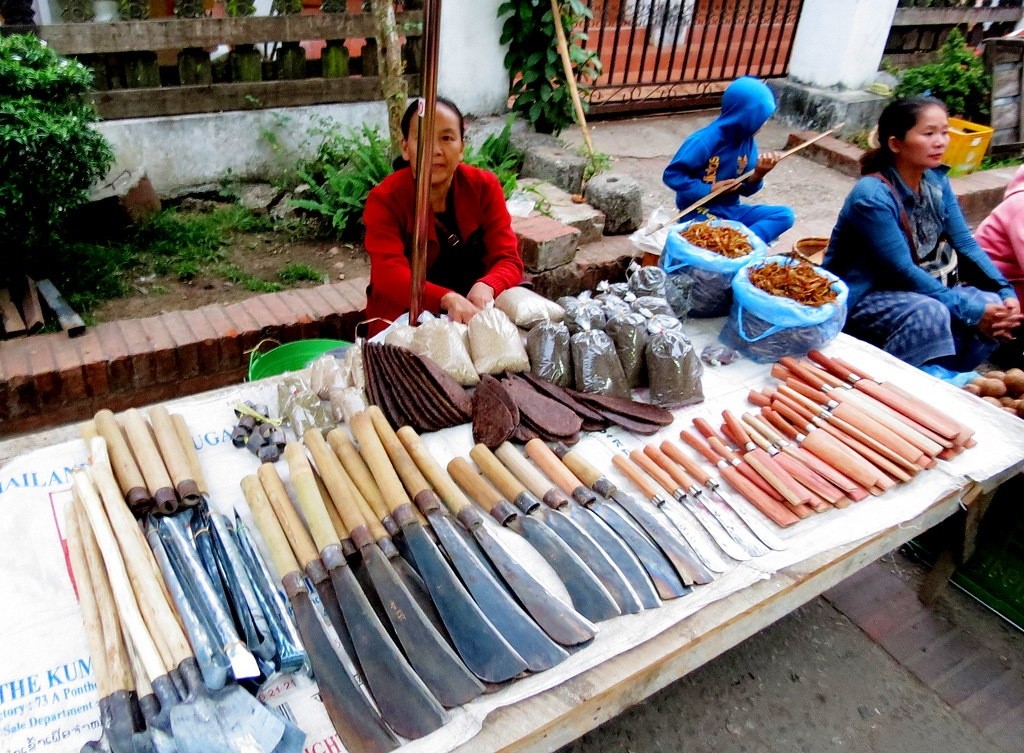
[[1, 290, 1024, 753]]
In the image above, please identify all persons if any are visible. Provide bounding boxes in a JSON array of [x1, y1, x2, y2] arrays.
[[363, 96, 524, 340], [662, 76, 795, 251], [820, 97, 1024, 373]]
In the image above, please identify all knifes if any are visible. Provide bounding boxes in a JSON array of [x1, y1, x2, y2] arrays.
[[240, 350, 979, 753]]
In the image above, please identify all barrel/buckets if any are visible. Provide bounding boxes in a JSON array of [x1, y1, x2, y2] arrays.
[[243, 338, 354, 383]]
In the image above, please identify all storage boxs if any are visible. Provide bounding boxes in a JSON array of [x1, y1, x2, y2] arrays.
[[942, 118, 995, 174]]
